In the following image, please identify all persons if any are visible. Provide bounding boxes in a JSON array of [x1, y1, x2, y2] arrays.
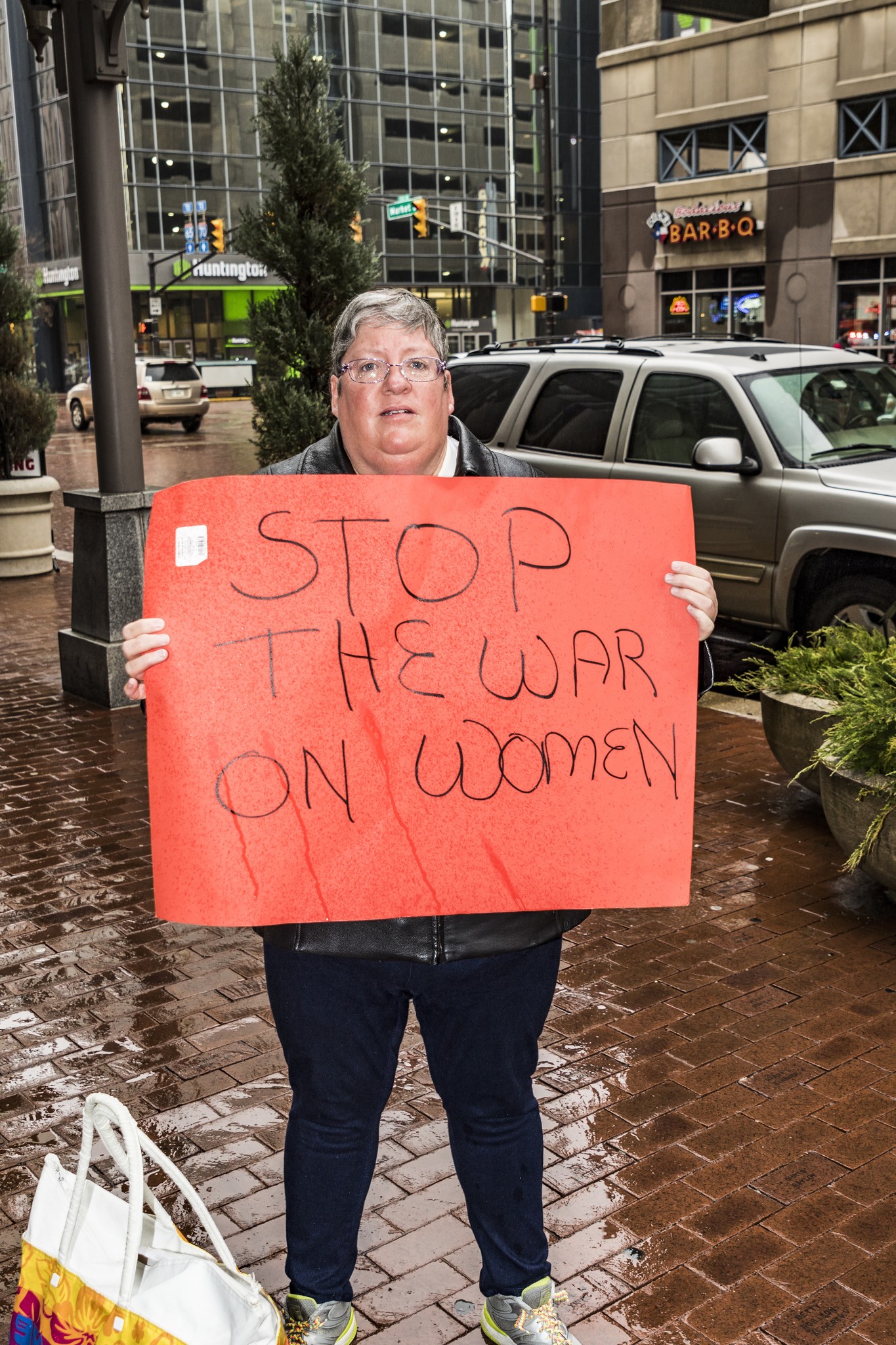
[[122, 288, 718, 1345]]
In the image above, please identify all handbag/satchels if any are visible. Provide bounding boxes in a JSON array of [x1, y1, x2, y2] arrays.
[[8, 1093, 290, 1345]]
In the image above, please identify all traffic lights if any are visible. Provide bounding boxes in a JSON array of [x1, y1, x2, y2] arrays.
[[264, 206, 278, 227], [411, 198, 428, 238], [139, 322, 155, 333], [348, 211, 364, 244], [209, 218, 224, 254], [530, 293, 568, 312]]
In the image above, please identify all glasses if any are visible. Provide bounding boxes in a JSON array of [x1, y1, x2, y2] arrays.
[[337, 357, 447, 385]]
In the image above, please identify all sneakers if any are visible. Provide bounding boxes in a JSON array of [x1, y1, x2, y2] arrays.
[[480, 1277, 583, 1345], [281, 1293, 357, 1345]]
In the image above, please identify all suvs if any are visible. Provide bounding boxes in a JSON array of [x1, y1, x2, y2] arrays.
[[442, 331, 895, 650], [65, 356, 211, 433]]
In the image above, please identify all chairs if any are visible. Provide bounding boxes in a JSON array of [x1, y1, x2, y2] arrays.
[[630, 397, 701, 464]]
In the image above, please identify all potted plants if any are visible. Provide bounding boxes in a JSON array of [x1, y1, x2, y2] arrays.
[[729, 615, 896, 901]]
